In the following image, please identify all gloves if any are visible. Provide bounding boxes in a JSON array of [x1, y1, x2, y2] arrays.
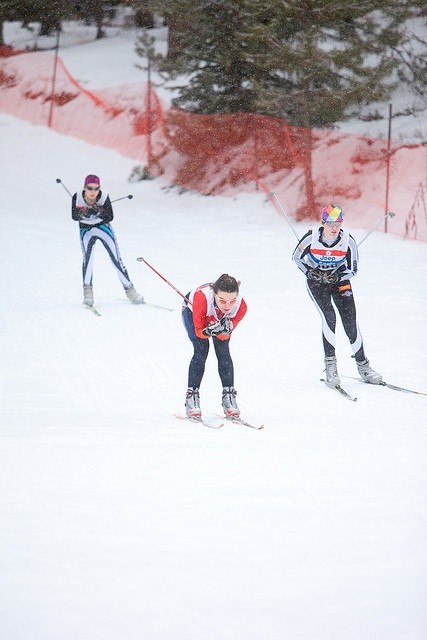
[[91, 204, 106, 219], [323, 271, 341, 284], [305, 270, 322, 282], [202, 320, 224, 337], [74, 207, 90, 220], [221, 317, 234, 334]]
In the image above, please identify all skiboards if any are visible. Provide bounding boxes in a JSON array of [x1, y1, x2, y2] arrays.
[[171, 413, 264, 430], [81, 301, 174, 316], [320, 372, 427, 402]]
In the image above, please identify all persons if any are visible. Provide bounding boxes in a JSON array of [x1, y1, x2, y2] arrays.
[[290, 203, 383, 387], [180, 273, 247, 421], [72, 175, 144, 306]]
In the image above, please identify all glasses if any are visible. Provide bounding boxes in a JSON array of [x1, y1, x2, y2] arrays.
[[214, 292, 238, 305], [324, 222, 342, 228], [83, 186, 100, 191]]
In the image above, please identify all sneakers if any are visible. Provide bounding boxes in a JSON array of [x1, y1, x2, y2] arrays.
[[185, 387, 201, 418], [125, 284, 144, 304], [356, 357, 382, 384], [322, 355, 341, 386], [222, 387, 240, 417], [82, 285, 94, 305]]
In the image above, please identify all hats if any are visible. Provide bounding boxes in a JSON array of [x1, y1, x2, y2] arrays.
[[321, 203, 343, 222], [84, 175, 100, 186]]
